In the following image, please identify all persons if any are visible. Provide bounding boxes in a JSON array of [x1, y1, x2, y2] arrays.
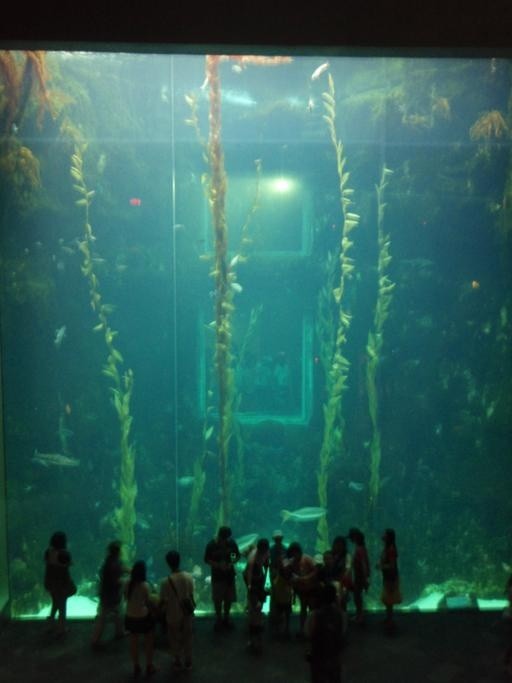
[[87, 539, 131, 649], [203, 521, 403, 681], [40, 530, 74, 638], [121, 561, 163, 676], [154, 550, 196, 673]]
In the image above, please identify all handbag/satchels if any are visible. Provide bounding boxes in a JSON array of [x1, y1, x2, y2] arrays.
[[179, 592, 198, 616]]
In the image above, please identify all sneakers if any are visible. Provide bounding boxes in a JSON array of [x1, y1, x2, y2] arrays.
[[89, 628, 131, 649], [213, 619, 321, 656], [132, 660, 195, 675], [46, 618, 72, 635]]
